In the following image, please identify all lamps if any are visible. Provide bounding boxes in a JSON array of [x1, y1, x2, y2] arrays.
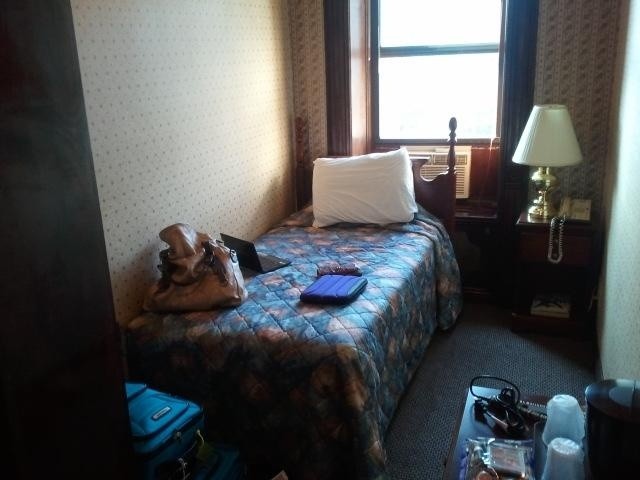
[[511, 103, 585, 220]]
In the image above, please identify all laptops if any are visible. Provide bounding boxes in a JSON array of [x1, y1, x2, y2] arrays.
[[220, 232, 291, 274]]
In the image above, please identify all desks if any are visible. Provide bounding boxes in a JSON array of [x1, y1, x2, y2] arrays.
[[443, 381, 583, 480]]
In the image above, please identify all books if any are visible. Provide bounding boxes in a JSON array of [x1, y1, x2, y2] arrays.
[[530, 292, 570, 318]]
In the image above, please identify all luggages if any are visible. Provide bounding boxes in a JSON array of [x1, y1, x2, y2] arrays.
[[126, 382, 206, 480]]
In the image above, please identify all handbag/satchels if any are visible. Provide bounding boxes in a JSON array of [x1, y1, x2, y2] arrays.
[[142, 223, 248, 312]]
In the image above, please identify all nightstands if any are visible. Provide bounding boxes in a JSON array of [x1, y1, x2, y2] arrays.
[[511, 205, 602, 341]]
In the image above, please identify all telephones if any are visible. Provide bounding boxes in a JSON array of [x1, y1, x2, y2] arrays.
[[559, 197, 592, 223]]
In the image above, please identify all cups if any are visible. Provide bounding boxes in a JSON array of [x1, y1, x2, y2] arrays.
[[542, 394, 585, 449], [540, 438, 585, 480]]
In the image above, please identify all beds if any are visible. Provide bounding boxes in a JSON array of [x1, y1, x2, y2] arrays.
[[122, 113, 458, 477]]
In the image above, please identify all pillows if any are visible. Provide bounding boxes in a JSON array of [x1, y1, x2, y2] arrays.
[[310, 146, 419, 229]]
